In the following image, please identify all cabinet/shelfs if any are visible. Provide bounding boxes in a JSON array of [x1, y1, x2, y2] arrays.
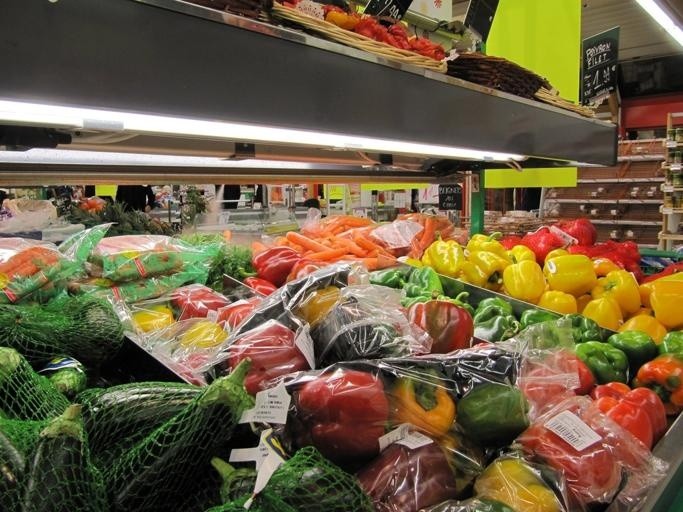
[[656, 111, 683, 251], [535, 138, 667, 255]]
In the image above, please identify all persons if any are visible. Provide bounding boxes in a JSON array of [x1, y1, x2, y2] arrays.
[[253, 185, 269, 208], [114, 184, 155, 215], [154, 185, 171, 209]]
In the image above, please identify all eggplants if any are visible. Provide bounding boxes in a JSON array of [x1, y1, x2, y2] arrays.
[[0, 295, 372, 512]]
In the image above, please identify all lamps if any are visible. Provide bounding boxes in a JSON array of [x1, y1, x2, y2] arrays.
[[636, 1, 683, 47], [0, 0, 616, 169]]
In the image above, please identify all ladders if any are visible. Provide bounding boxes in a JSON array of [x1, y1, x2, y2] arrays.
[[326, 182, 350, 216]]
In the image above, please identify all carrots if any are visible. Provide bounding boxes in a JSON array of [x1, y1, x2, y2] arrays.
[[0, 246, 57, 281]]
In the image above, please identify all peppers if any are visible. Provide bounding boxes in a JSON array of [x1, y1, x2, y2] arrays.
[[133, 214, 683, 512], [323, 6, 445, 61]]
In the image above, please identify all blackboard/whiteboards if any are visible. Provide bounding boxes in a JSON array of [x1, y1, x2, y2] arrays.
[[439, 183, 462, 210]]
